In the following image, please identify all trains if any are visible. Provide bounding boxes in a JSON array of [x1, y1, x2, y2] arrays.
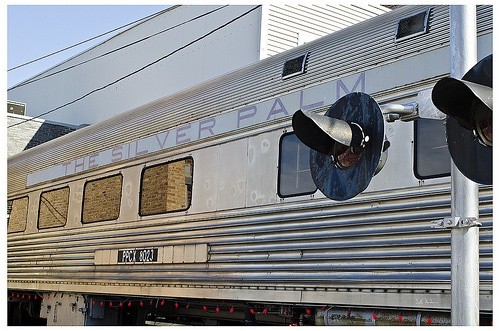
[[6, 4, 492, 327]]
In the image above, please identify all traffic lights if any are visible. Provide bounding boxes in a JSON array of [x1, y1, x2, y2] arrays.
[[431, 54, 492, 186], [292, 92, 384, 201]]
